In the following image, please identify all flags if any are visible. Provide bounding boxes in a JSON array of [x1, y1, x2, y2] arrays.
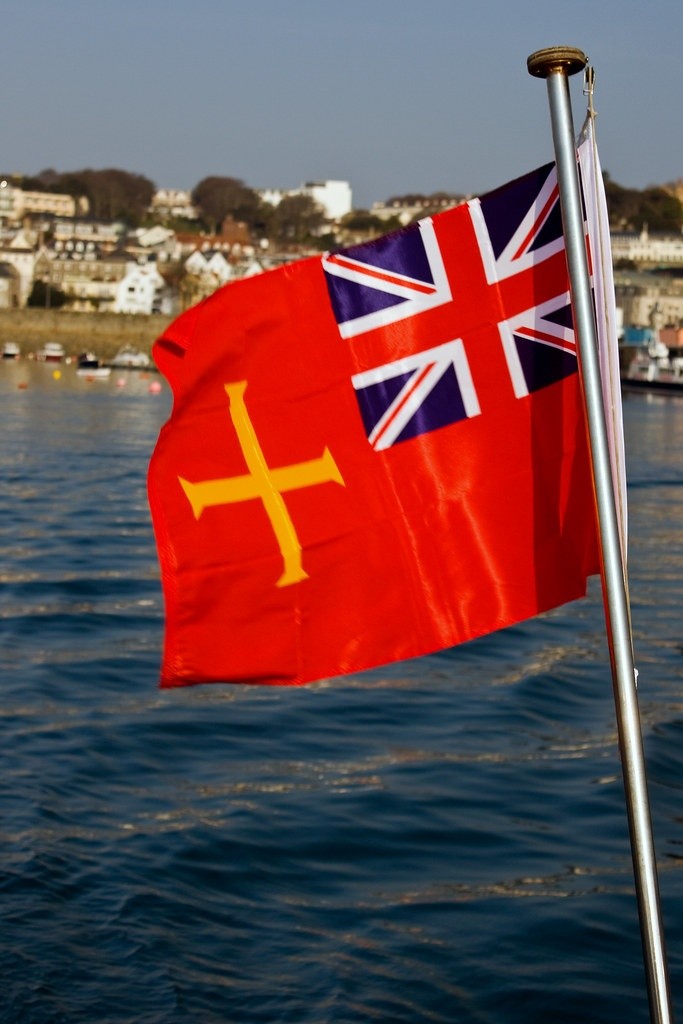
[[145, 124, 622, 690]]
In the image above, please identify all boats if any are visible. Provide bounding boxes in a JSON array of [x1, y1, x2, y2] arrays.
[[101, 342, 158, 373], [620, 340, 683, 397], [36, 342, 65, 363], [1, 342, 20, 358], [75, 352, 110, 378]]
